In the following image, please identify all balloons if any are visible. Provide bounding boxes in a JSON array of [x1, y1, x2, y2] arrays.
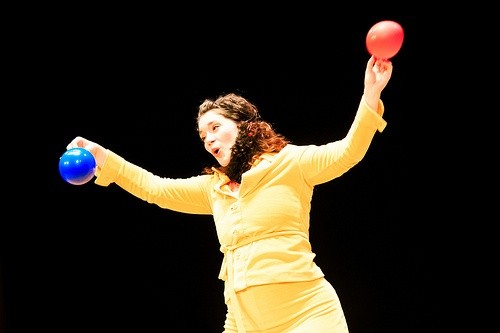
[[366, 21, 404, 61], [60, 148, 97, 186]]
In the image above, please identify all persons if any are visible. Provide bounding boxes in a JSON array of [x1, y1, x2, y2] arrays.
[[66, 56, 392, 333]]
